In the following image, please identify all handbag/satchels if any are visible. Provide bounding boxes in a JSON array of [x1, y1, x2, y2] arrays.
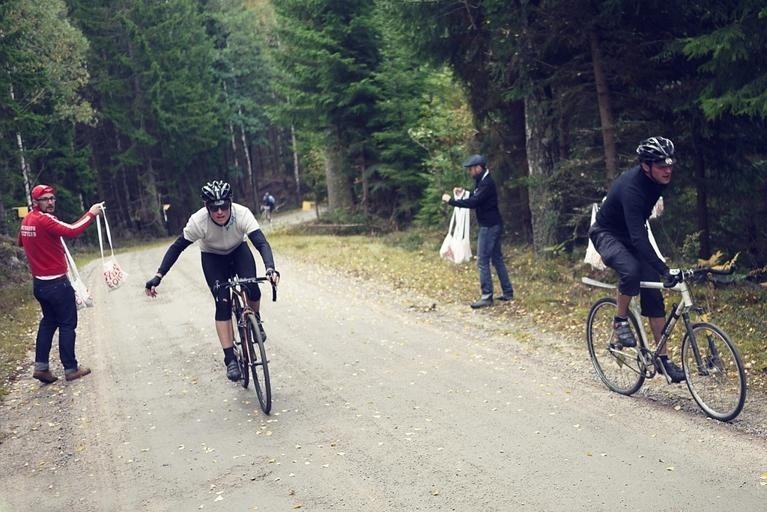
[[102, 254, 127, 292], [70, 274, 93, 309], [440, 231, 472, 264]]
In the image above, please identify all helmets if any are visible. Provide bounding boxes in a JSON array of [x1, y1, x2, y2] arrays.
[[637, 136, 677, 168], [201, 180, 232, 206]]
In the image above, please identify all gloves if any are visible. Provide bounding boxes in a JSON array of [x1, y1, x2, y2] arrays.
[[663, 268, 683, 286]]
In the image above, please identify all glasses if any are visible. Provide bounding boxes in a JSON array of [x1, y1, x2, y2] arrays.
[[209, 204, 229, 212], [37, 196, 57, 202]]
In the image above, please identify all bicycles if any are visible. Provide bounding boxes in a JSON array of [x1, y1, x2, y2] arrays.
[[577, 262, 748, 422], [209, 271, 280, 415], [258, 205, 272, 225]]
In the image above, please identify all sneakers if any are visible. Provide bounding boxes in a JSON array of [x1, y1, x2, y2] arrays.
[[253, 321, 266, 343], [226, 360, 240, 382], [34, 370, 57, 383], [658, 357, 686, 381], [496, 295, 511, 301], [614, 316, 637, 347], [66, 366, 91, 381], [471, 298, 494, 308]]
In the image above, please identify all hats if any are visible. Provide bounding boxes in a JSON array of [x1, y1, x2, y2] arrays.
[[31, 184, 55, 199], [464, 155, 485, 168]]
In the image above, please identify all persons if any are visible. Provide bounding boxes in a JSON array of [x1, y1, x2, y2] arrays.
[[17, 185, 106, 384], [260, 191, 275, 223], [442, 154, 513, 309], [589, 136, 686, 383], [145, 180, 280, 381]]
